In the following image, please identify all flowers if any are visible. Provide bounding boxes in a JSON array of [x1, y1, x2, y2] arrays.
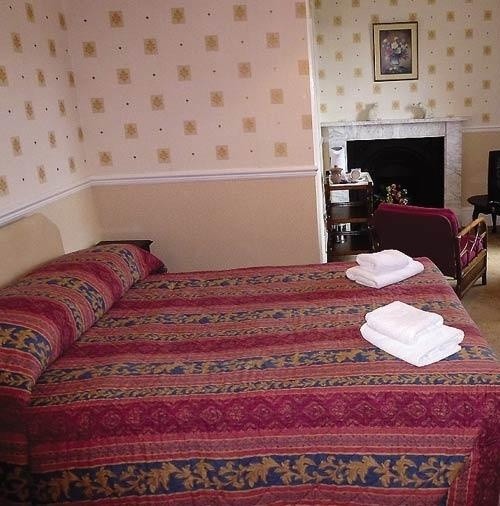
[[374, 183, 409, 205]]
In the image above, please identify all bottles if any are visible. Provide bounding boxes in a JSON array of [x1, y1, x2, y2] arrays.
[[330, 164, 343, 184], [332, 224, 347, 244]]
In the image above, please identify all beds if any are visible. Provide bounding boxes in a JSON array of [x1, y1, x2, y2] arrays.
[[1, 243, 500, 506]]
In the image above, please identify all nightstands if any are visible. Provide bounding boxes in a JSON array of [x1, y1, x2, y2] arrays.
[[95, 239, 154, 254]]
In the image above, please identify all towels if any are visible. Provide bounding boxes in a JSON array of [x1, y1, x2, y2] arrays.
[[364, 300, 444, 346], [345, 260, 425, 289], [360, 324, 465, 367], [356, 249, 410, 275]]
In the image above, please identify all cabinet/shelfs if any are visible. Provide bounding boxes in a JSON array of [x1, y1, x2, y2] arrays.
[[324, 170, 375, 262]]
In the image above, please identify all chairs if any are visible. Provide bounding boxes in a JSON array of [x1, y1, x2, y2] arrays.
[[467, 149, 500, 233], [375, 203, 487, 300]]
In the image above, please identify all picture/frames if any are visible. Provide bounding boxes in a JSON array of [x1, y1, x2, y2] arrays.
[[373, 21, 418, 82]]
